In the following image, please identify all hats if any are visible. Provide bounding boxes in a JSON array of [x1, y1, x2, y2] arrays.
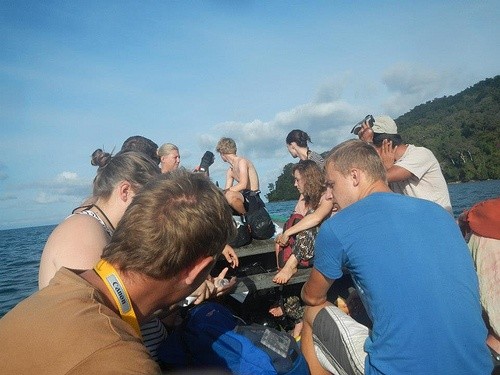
[[372, 115, 397, 135]]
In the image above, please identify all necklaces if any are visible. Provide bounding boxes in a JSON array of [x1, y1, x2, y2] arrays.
[[72, 204, 116, 231]]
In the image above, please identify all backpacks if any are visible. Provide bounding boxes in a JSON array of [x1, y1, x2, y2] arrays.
[[156, 302, 311, 375]]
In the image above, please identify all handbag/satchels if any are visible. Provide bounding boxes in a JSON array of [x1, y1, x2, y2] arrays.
[[235, 224, 253, 248], [462, 197, 500, 240], [245, 206, 275, 241]]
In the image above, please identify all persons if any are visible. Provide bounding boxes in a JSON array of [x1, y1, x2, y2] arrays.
[[156, 143, 180, 173], [40, 149, 236, 359], [351, 115, 454, 211], [286, 130, 324, 168], [122, 135, 239, 270], [300, 139, 494, 375], [216, 138, 259, 217], [270, 159, 339, 316], [0, 171, 233, 375]]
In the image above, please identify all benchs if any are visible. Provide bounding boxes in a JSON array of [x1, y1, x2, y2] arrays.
[[232, 238, 276, 257], [230, 267, 314, 294]]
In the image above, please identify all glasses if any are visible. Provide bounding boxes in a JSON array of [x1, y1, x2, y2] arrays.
[[350, 114, 375, 135]]
